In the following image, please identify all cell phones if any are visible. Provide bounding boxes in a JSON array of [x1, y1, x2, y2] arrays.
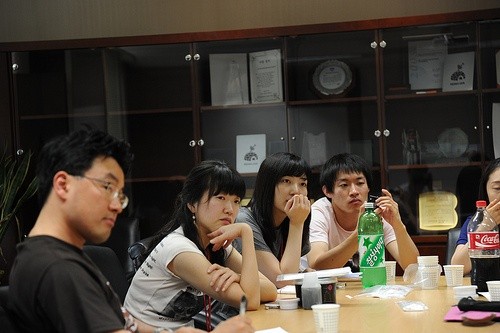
[[366, 195, 379, 211]]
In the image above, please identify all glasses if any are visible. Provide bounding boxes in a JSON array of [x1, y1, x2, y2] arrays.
[[77, 175, 128, 209]]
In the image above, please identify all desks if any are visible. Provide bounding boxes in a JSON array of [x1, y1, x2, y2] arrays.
[[246, 277, 500, 333]]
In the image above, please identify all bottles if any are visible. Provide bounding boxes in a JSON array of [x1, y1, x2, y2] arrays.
[[301, 272, 322, 310], [357, 202, 386, 287], [467, 201, 500, 295]]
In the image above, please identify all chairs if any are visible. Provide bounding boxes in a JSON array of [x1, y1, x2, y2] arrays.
[[83, 216, 162, 299]]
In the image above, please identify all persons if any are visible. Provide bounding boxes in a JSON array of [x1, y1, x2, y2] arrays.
[[451, 158, 500, 275], [5, 122, 258, 333], [300, 153, 420, 272], [231, 152, 311, 287], [124, 159, 277, 329]]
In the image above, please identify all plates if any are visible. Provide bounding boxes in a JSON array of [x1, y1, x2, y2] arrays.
[[312, 60, 352, 95]]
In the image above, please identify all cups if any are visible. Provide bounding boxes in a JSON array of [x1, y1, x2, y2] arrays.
[[453, 286, 477, 306], [311, 303, 340, 333], [386, 260, 397, 281], [443, 264, 465, 287], [417, 255, 440, 289], [486, 281, 500, 301]]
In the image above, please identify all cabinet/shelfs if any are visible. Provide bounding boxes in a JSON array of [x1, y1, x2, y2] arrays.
[[0, 8, 500, 243]]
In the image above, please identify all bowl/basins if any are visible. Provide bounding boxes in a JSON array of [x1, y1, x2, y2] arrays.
[[294, 277, 339, 309]]
